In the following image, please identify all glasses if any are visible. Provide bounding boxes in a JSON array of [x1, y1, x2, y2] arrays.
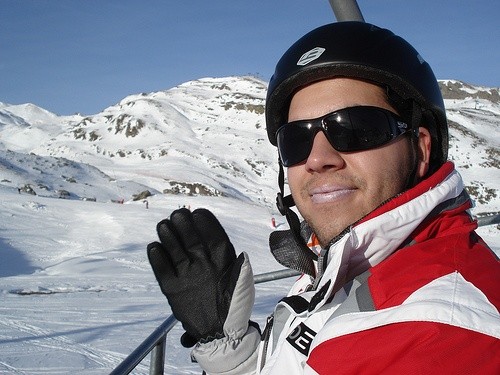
[[275, 105, 418, 167]]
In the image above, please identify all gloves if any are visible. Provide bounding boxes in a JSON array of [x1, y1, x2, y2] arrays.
[[146, 206, 261, 375]]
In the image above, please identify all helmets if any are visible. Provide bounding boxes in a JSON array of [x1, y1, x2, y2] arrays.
[[265, 21, 449, 160]]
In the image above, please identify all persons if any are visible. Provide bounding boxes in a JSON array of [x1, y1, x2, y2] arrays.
[[145, 20, 500, 375]]
